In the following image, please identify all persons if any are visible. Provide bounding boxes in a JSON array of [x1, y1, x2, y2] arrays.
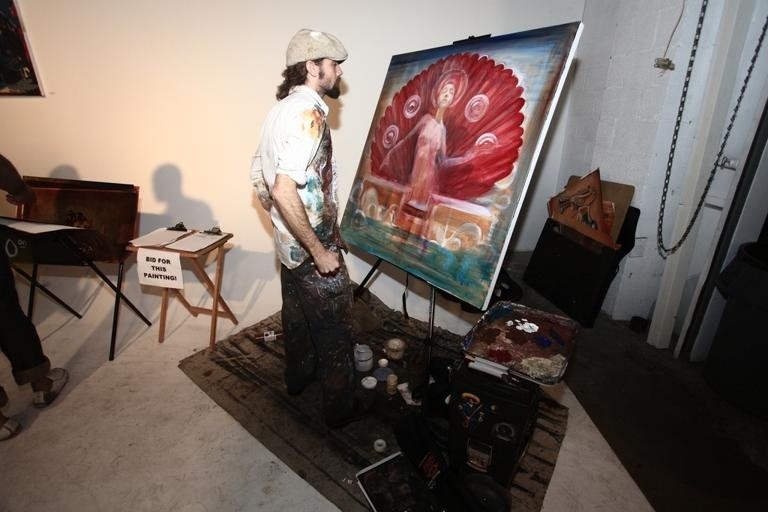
[[247, 27, 358, 443], [377, 80, 501, 246], [0, 154, 69, 441]]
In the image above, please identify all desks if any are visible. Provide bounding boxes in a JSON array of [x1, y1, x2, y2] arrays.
[[126, 233, 235, 353]]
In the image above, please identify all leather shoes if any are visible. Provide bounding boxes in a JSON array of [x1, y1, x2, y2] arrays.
[[32, 367, 68, 408], [287, 373, 306, 396], [325, 416, 335, 432], [0, 416, 22, 441]]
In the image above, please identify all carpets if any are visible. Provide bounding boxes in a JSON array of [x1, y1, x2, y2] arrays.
[[177, 279, 569, 510]]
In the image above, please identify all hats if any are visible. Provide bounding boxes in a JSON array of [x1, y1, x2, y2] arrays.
[[286, 28, 348, 67]]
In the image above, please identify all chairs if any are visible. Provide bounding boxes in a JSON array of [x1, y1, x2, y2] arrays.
[[521, 205, 640, 328]]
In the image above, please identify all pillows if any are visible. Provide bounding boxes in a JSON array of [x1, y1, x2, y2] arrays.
[[547, 167, 635, 251]]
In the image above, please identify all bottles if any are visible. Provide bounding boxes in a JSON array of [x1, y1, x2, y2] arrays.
[[353, 344, 374, 388], [372, 358, 394, 394], [254, 330, 284, 344]]
